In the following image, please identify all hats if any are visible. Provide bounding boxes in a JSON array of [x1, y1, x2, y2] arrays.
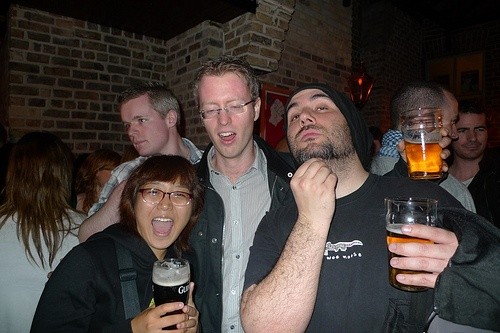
[[285, 83, 373, 172]]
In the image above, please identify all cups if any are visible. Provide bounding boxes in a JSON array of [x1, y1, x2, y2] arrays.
[[400, 108, 442, 180], [153, 259, 190, 330], [384, 198, 438, 291]]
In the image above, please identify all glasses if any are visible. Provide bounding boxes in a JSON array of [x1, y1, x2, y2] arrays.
[[199, 99, 257, 119], [137, 188, 194, 206]]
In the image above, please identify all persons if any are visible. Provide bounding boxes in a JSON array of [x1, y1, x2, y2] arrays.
[[0, 56, 500, 332]]
